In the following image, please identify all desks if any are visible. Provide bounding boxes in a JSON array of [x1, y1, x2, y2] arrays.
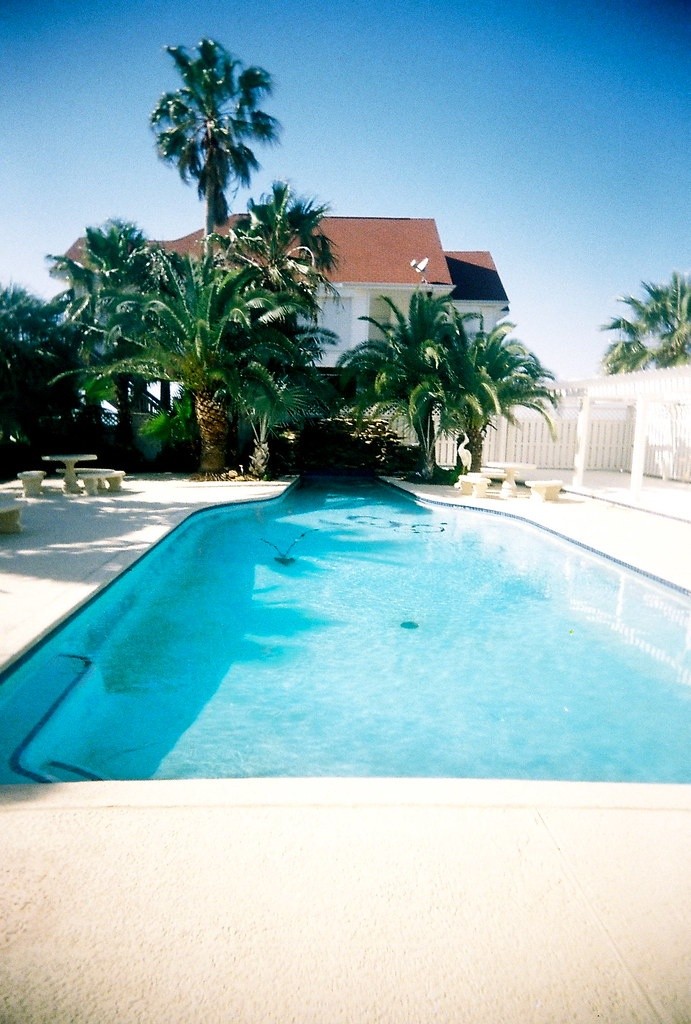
[[486, 463, 537, 498], [42, 454, 97, 493]]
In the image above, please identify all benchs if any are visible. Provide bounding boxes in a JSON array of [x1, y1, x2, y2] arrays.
[[457, 469, 563, 500], [17, 468, 125, 497]]
